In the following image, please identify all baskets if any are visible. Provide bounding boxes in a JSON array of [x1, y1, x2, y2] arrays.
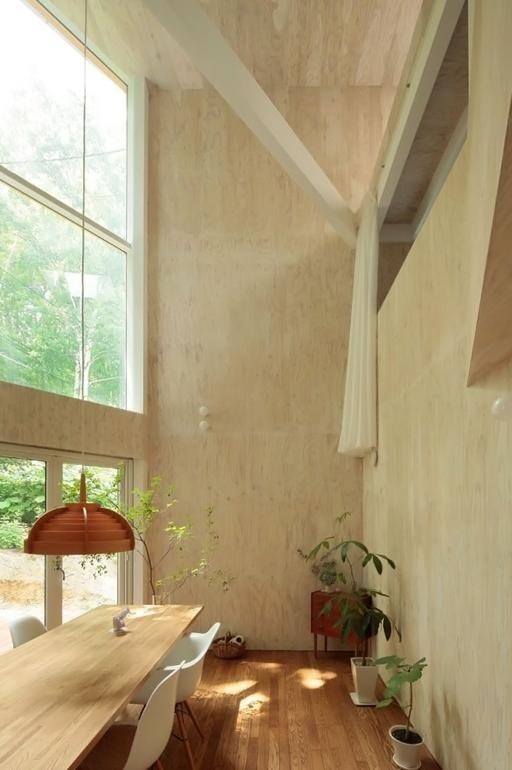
[[211, 631, 246, 659]]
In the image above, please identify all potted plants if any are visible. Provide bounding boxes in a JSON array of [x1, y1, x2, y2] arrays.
[[293, 508, 430, 770]]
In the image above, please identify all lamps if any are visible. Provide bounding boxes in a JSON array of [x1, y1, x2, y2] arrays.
[[22, 2, 138, 561]]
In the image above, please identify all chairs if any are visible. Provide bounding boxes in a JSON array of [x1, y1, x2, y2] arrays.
[[8, 615, 47, 647], [82, 619, 221, 770]]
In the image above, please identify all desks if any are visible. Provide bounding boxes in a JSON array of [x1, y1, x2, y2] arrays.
[[0, 602, 205, 770]]
